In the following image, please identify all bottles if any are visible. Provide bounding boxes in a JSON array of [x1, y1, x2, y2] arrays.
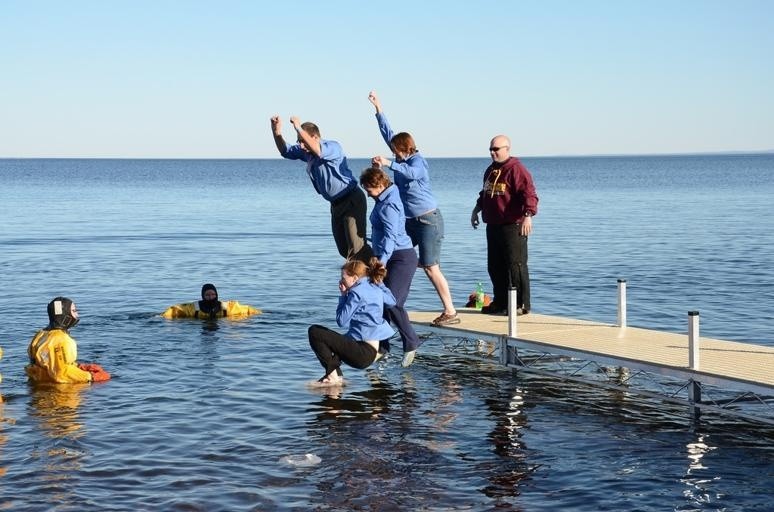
[[476, 282, 484, 310]]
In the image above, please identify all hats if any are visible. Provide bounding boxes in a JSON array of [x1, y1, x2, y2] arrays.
[[46, 298, 79, 331], [202, 284, 219, 307]]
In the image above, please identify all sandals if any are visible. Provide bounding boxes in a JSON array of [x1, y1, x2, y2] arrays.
[[432, 312, 460, 326]]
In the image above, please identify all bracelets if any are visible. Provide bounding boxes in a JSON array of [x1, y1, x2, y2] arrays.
[[295, 128, 303, 132], [390, 160, 392, 168]]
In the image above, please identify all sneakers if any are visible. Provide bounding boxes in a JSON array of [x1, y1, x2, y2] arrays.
[[482, 303, 530, 317]]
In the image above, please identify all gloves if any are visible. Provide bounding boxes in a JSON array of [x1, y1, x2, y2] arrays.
[[77, 363, 110, 381]]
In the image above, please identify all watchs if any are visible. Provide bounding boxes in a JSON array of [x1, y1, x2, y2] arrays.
[[523, 212, 532, 216]]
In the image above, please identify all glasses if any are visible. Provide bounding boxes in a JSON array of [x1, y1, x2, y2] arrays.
[[489, 146, 509, 152]]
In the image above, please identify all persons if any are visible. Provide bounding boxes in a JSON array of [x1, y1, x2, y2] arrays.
[[308, 256, 396, 389], [470, 135, 539, 315], [360, 158, 419, 368], [369, 90, 462, 327], [270, 113, 368, 258], [163, 283, 261, 320], [27, 297, 111, 383]]
